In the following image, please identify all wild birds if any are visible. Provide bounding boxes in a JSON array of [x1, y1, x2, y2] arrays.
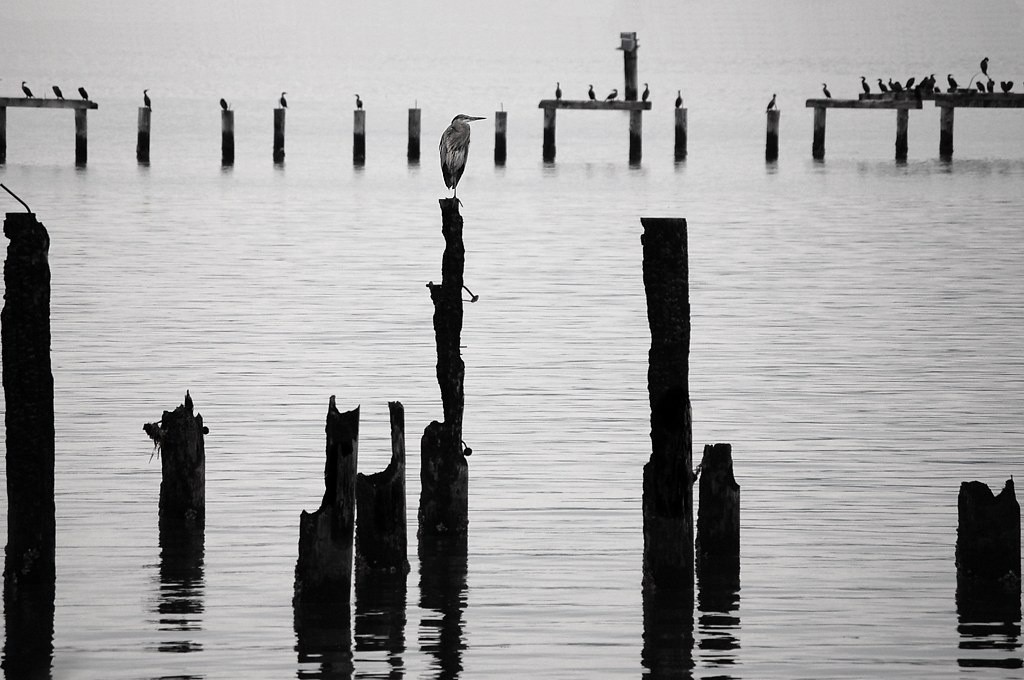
[[822, 83, 832, 99], [876, 79, 888, 93], [78, 87, 88, 100], [889, 77, 915, 92], [355, 94, 362, 110], [439, 115, 486, 198], [920, 74, 936, 89], [144, 89, 150, 109], [675, 90, 682, 108], [556, 82, 562, 100], [52, 86, 65, 100], [220, 99, 227, 110], [860, 76, 870, 94], [642, 83, 649, 102], [948, 57, 1014, 93], [281, 92, 288, 108], [588, 85, 597, 101], [766, 94, 776, 113], [604, 89, 618, 102], [22, 81, 33, 98]]
